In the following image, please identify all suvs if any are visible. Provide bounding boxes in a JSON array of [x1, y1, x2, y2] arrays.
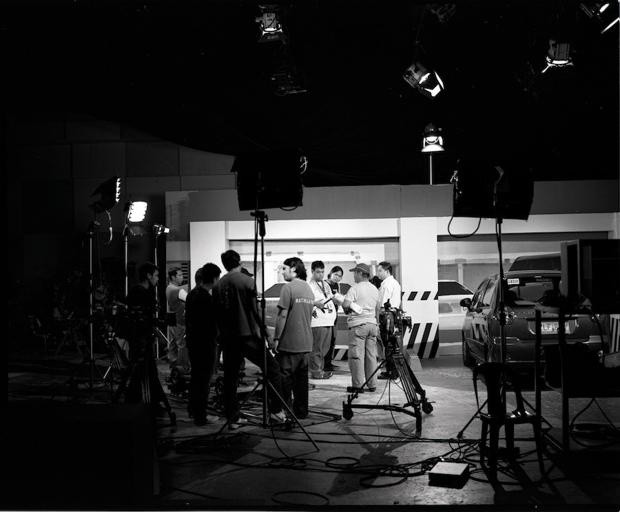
[[438, 280, 476, 334]]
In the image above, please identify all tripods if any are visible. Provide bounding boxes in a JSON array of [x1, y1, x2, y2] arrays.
[[48, 273, 117, 405], [214, 237, 319, 452], [455, 256, 554, 440]]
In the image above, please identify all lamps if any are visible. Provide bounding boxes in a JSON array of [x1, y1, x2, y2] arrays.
[[420, 123, 447, 154], [581, 0, 620, 34], [103, 200, 148, 378], [220, 143, 319, 454], [404, 64, 445, 99], [451, 147, 555, 448], [541, 36, 575, 74], [154, 225, 169, 359], [255, 12, 286, 42], [89, 178, 121, 378]]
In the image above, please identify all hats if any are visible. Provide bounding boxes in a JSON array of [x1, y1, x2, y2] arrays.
[[241, 267, 253, 277], [349, 262, 371, 275]]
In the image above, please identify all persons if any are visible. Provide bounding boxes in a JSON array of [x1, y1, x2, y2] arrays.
[[123, 250, 400, 425]]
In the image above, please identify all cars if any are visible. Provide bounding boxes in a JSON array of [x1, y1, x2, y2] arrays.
[[256, 282, 352, 329]]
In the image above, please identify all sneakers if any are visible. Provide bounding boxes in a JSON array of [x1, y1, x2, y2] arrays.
[[270, 411, 297, 424], [369, 387, 377, 393], [194, 414, 220, 426], [346, 386, 365, 393], [228, 418, 248, 430], [312, 371, 333, 379], [377, 372, 399, 380]]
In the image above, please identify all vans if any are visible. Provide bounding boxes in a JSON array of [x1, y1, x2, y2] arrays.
[[459, 268, 607, 381], [505, 250, 562, 300]]
